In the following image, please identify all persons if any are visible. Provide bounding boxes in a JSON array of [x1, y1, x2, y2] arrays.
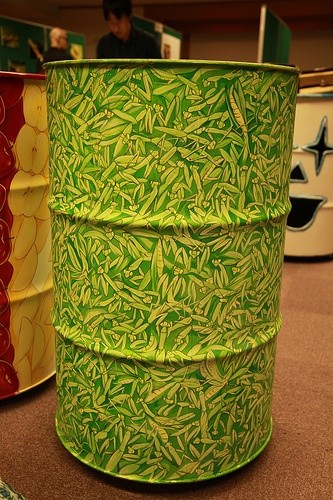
[[37, 26, 74, 74], [96, 0, 163, 59]]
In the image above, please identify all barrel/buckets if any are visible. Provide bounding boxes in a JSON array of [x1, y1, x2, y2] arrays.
[[284, 90, 332, 258], [1, 72, 59, 399], [44, 58, 299, 485]]
